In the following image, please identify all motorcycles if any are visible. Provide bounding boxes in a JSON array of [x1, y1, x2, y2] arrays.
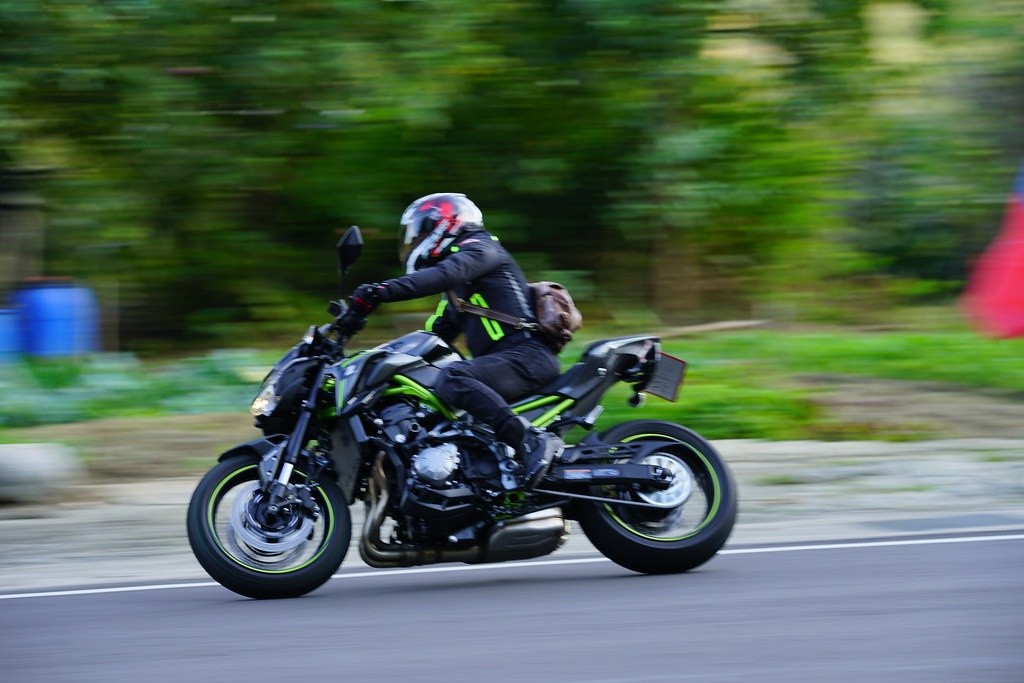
[[185, 223, 738, 601]]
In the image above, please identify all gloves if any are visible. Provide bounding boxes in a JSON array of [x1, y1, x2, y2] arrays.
[[353, 282, 389, 315]]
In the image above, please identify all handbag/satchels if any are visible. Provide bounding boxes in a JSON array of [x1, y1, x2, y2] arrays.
[[528, 281, 583, 353]]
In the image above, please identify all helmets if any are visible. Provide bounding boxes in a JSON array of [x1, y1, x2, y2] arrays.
[[400, 192, 484, 275]]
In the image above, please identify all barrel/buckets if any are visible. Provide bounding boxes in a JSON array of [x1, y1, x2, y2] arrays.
[[12, 276, 101, 360]]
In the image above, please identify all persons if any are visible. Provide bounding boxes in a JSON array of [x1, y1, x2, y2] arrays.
[[353, 193, 559, 491]]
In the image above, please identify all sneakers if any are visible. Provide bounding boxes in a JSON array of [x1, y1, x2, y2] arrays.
[[517, 426, 563, 492]]
